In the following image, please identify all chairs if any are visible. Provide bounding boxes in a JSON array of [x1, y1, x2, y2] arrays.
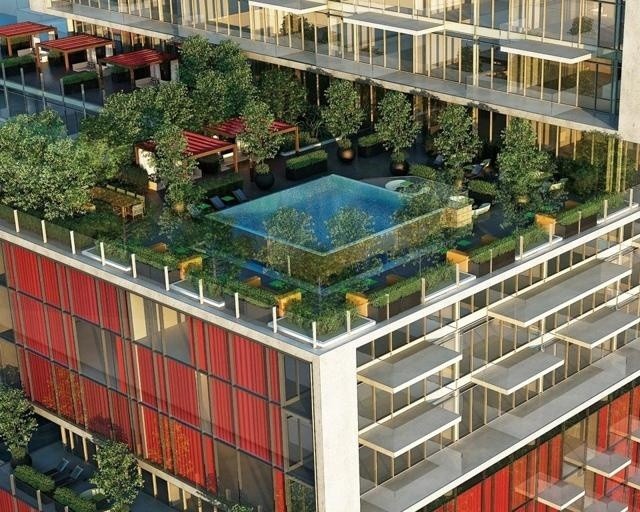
[[84, 182, 147, 223], [55, 466, 85, 487], [45, 457, 71, 477]]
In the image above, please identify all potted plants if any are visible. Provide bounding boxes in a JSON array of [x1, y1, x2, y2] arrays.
[[320, 78, 367, 162], [492, 115, 548, 214], [372, 89, 423, 175], [432, 97, 481, 191], [0, 379, 37, 472], [150, 126, 199, 216]]
